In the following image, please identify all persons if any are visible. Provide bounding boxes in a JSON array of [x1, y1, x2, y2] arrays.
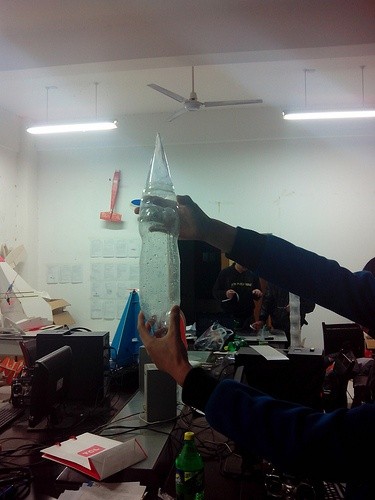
[[210, 260, 263, 333], [252, 281, 315, 350], [135, 195, 375, 500], [354, 257, 375, 340]]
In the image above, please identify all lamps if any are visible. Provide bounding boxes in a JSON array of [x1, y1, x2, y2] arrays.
[[27, 81, 118, 136], [283, 65, 375, 121]]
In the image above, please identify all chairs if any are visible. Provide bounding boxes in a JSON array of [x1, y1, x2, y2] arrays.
[[322, 322, 364, 359]]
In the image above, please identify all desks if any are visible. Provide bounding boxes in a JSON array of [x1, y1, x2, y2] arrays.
[[0, 348, 263, 499], [181, 325, 288, 345]]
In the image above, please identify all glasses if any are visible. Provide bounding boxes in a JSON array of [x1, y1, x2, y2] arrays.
[[263, 473, 316, 500]]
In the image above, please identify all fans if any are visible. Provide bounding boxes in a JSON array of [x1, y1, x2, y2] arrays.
[[145, 66, 264, 123]]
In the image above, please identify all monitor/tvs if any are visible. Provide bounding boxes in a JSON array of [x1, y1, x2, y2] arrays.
[[232, 354, 324, 412], [19, 324, 69, 366], [29, 345, 72, 427]]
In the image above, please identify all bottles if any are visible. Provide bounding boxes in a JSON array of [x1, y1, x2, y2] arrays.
[[175, 430, 206, 500], [224, 340, 245, 350]]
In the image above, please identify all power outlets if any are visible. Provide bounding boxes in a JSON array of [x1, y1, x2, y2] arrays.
[[47, 272, 58, 284]]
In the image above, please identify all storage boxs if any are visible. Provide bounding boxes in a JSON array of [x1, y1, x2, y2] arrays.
[[47, 299, 76, 326]]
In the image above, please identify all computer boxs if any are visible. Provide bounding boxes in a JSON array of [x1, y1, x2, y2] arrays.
[[36, 330, 111, 408]]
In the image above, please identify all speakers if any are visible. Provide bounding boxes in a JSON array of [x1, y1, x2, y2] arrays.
[[144, 364, 177, 426], [139, 345, 154, 392]]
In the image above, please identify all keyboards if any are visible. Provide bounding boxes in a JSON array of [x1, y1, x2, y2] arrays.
[[0, 408, 24, 432], [322, 481, 345, 500]]
